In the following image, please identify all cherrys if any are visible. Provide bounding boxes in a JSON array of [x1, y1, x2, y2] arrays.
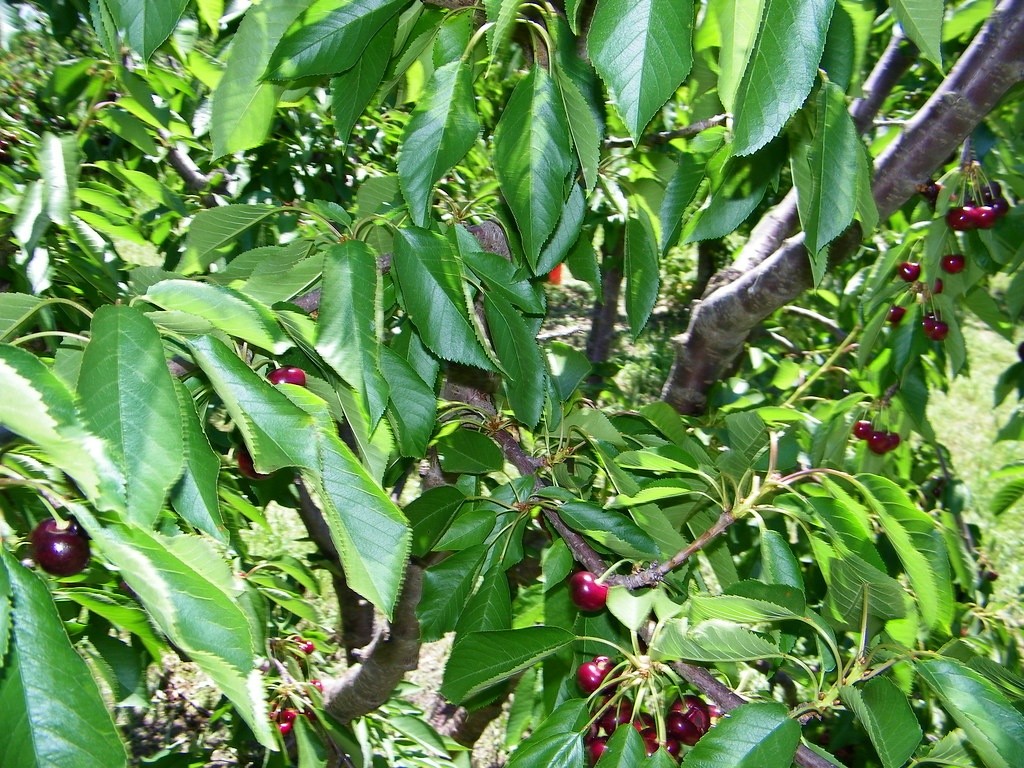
[[0, 157, 1009, 765]]
